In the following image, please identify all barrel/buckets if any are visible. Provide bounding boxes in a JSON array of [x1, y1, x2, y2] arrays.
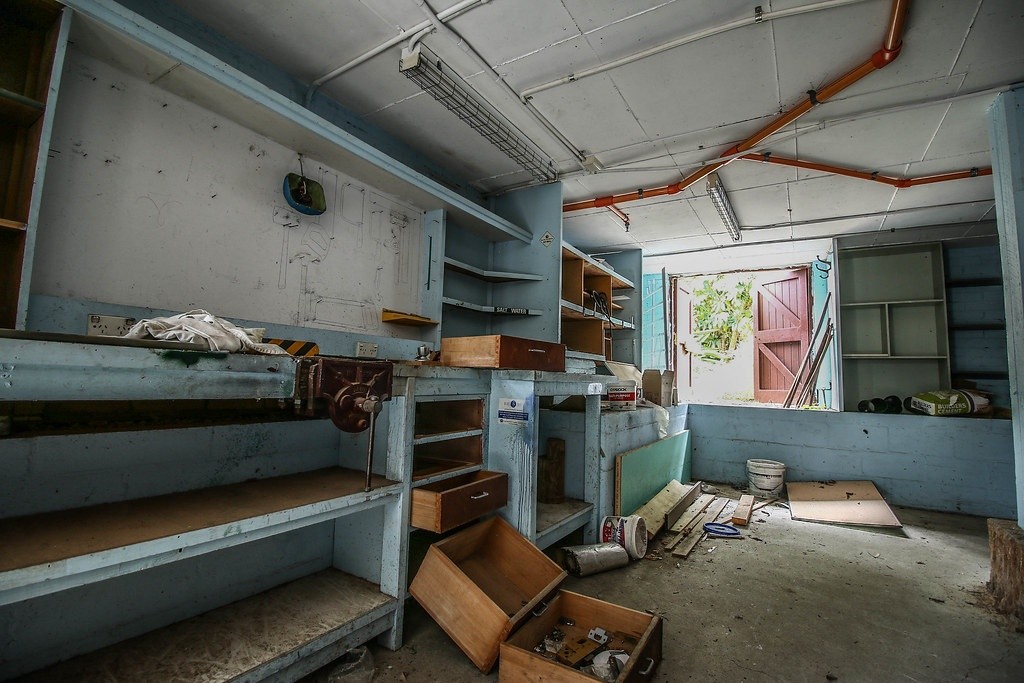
[[606, 380, 638, 412], [599, 513, 649, 562], [744, 459, 786, 500]]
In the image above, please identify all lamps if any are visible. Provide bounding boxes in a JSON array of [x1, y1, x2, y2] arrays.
[[398, 42, 558, 183], [705, 173, 741, 243]]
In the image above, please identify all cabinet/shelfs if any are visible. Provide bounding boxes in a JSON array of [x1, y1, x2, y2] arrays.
[[839, 234, 1012, 413], [0, 0, 688, 683]]
